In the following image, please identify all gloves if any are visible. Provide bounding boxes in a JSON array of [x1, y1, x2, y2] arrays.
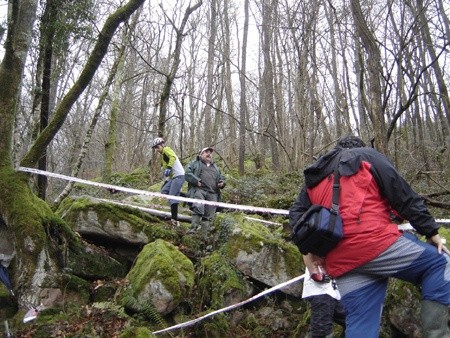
[[165, 169, 171, 176]]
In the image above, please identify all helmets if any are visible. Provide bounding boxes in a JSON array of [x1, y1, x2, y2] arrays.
[[151, 137, 165, 149]]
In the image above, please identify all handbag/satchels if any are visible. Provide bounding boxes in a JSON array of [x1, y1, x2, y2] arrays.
[[290, 204, 344, 257]]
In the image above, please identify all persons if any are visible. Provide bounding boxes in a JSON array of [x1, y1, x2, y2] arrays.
[[288, 136, 450, 338], [152, 137, 186, 226], [184, 147, 227, 235]]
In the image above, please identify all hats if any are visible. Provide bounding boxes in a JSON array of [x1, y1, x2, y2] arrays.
[[199, 147, 213, 155]]
